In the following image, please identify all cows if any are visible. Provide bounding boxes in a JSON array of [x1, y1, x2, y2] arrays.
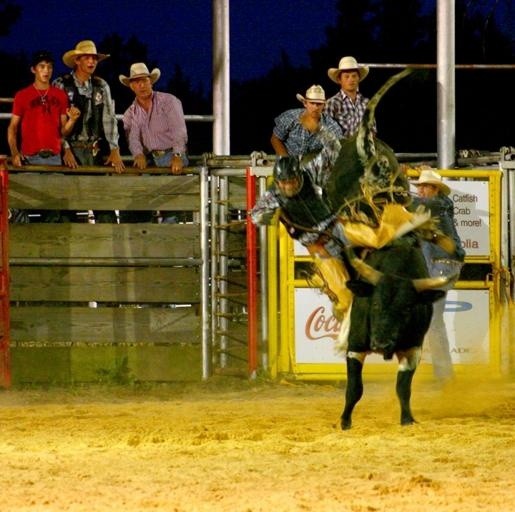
[[322, 64, 458, 431]]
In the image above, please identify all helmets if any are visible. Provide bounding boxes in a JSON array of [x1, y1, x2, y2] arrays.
[[273, 156, 302, 181]]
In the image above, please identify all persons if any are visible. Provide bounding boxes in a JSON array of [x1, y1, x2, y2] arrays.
[[117, 62, 191, 224], [250, 113, 355, 312], [405, 169, 468, 395], [321, 55, 379, 140], [270, 85, 343, 185], [51, 40, 128, 225], [6, 55, 84, 224]]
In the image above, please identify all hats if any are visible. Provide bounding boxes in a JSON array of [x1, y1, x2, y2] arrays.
[[410, 169, 451, 196], [118, 62, 162, 87], [62, 40, 111, 69], [327, 55, 370, 83], [296, 84, 328, 104]]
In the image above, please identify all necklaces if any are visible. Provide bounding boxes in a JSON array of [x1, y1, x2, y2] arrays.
[[32, 83, 49, 104]]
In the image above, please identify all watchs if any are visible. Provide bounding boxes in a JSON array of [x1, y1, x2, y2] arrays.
[[173, 153, 184, 159]]
